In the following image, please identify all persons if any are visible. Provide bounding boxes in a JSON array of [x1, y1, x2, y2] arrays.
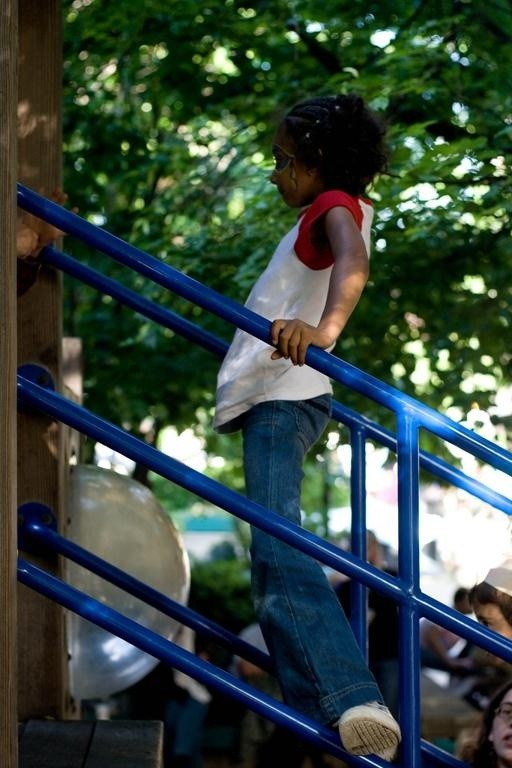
[[214, 92, 405, 768], [95, 559, 511, 766]]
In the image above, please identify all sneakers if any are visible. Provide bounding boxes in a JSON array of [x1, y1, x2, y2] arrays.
[[336, 696, 405, 764]]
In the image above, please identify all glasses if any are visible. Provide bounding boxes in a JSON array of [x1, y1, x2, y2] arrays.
[[493, 706, 512, 720]]
